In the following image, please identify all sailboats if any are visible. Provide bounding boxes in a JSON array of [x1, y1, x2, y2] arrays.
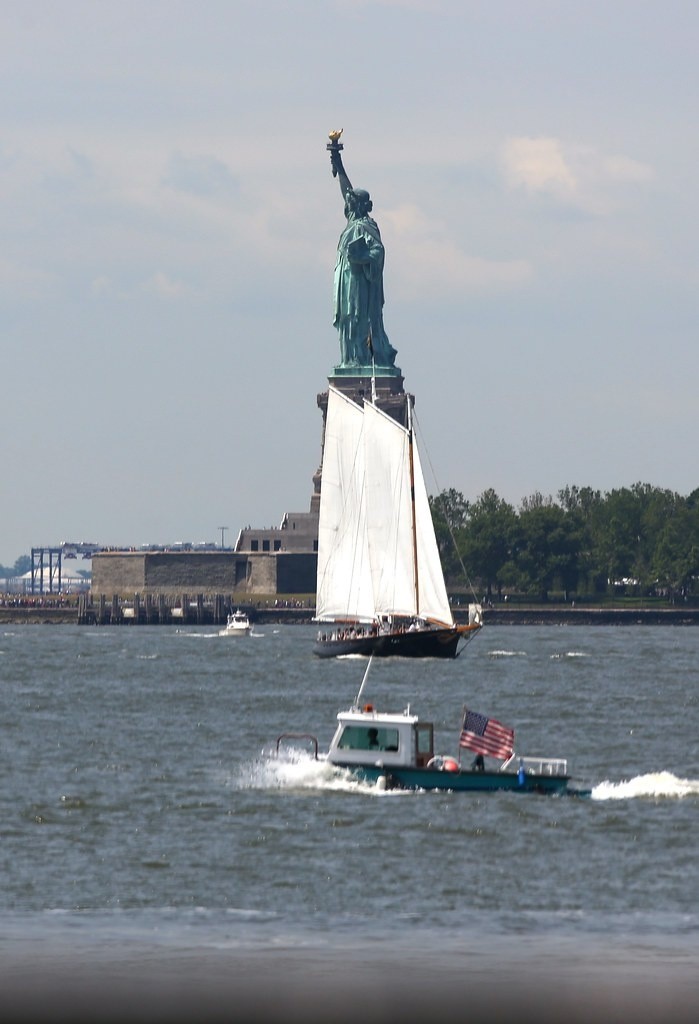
[[311, 385, 483, 659]]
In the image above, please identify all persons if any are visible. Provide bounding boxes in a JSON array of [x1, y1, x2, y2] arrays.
[[323, 619, 430, 642], [331, 151, 400, 367], [367, 729, 380, 748], [257, 597, 302, 608], [0, 597, 72, 608]]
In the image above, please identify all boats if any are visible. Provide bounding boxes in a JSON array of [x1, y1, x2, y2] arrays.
[[218, 611, 252, 636], [259, 704, 592, 799]]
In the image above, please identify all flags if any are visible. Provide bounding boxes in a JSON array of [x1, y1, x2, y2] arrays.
[[460, 709, 514, 759]]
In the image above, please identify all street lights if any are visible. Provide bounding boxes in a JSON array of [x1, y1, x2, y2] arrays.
[[219, 527, 227, 548]]
